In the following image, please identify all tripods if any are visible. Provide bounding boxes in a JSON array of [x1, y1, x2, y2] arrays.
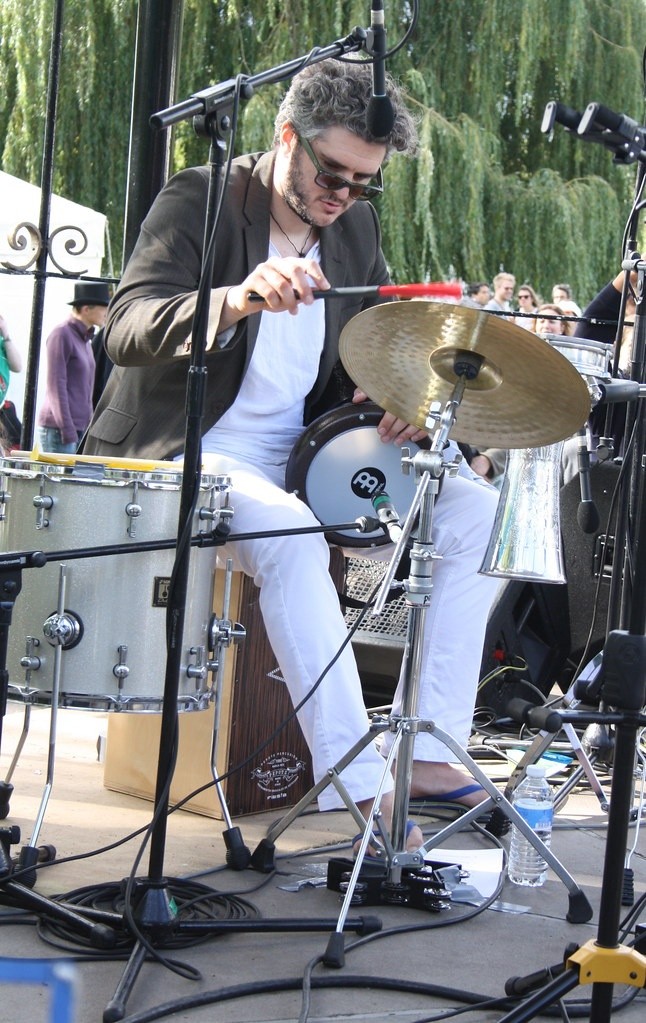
[[0, 29, 378, 1021], [255, 368, 603, 943]]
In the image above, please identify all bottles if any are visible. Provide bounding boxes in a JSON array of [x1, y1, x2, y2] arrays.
[[508, 763, 555, 887]]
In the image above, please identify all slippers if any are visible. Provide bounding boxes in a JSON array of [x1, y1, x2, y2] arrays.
[[409, 783, 497, 823], [352, 821, 424, 869]]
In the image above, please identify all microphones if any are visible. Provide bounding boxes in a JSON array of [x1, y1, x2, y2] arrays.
[[578, 445, 600, 533], [370, 490, 403, 542], [367, 0, 396, 136]]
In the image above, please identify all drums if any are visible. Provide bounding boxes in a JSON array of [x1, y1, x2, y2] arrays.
[[475, 332, 612, 586], [0, 448, 231, 714]]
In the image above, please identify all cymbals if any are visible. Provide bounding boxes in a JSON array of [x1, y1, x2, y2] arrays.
[[337, 300, 590, 449]]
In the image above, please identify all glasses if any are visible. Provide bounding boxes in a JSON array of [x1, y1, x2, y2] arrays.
[[298, 136, 384, 201], [517, 295, 531, 299]]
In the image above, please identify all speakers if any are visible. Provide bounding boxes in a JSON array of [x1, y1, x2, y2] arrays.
[[559, 416, 646, 655], [340, 542, 565, 716], [104, 544, 346, 818]]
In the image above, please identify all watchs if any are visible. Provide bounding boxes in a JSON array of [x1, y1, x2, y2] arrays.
[[2, 335, 12, 342]]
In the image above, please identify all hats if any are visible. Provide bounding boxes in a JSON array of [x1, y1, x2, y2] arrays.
[[67, 282, 110, 306]]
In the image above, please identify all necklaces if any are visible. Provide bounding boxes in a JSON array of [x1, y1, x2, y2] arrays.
[[268, 211, 313, 258]]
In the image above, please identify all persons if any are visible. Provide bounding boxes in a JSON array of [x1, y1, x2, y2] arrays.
[[76, 51, 506, 852], [0, 311, 21, 458], [407, 267, 646, 730], [38, 281, 109, 453], [93, 328, 115, 418]]
